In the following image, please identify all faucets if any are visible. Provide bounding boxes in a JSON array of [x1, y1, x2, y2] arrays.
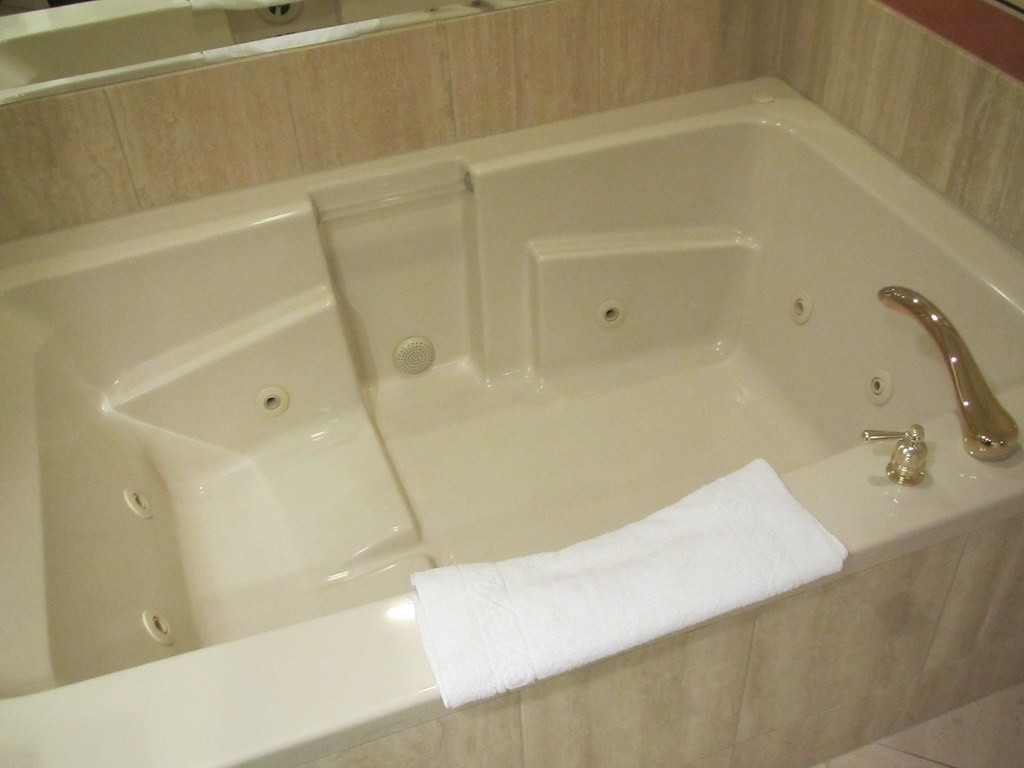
[[877, 285, 1021, 462]]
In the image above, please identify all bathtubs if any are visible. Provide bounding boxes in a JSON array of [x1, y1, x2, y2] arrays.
[[1, 74, 1024, 768]]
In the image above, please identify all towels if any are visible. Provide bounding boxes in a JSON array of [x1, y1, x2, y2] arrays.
[[409, 457, 851, 699]]
